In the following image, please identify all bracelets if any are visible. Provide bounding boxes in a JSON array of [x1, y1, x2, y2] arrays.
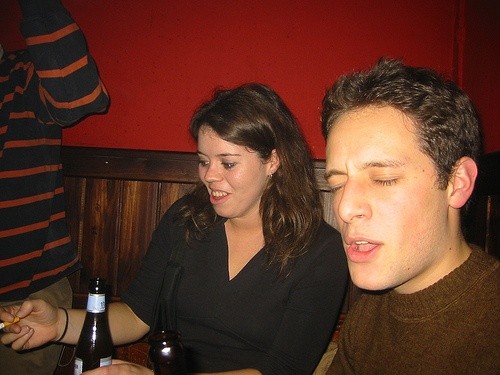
[[55, 307, 69, 342]]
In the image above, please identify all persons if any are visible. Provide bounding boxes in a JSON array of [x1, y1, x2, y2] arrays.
[[321, 55, 500, 375], [0, 0, 110, 375], [0, 82, 350, 375]]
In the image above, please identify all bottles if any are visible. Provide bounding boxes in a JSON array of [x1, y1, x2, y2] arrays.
[[147, 328, 191, 375], [73, 276, 112, 375]]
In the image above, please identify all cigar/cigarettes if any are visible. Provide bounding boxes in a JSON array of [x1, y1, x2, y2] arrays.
[[0, 316, 19, 329]]
